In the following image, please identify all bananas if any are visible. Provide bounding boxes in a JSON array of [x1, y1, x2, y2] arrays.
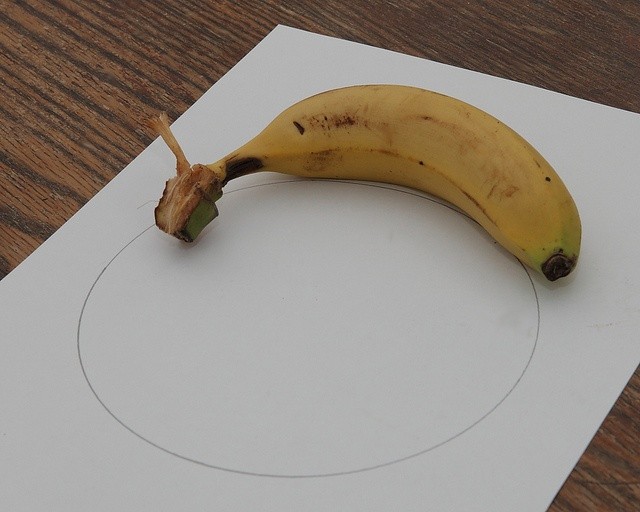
[[147, 84, 582, 282]]
